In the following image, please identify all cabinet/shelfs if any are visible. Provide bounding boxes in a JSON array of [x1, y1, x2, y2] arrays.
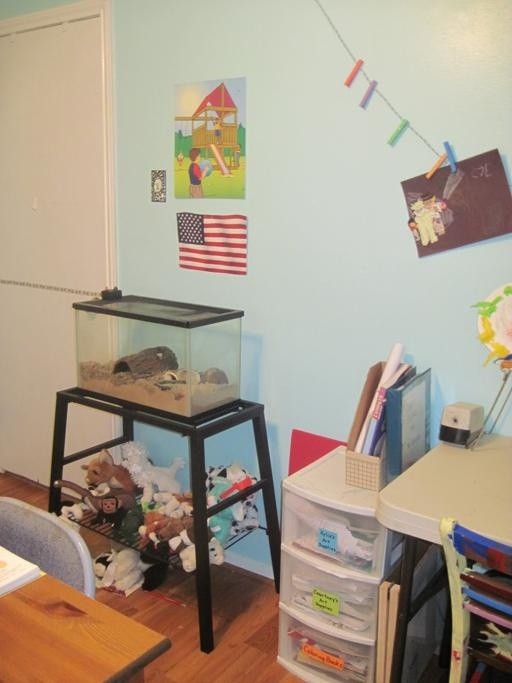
[[277, 445, 404, 682]]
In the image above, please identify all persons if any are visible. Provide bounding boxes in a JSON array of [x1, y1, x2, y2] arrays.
[[212, 115, 224, 145], [234, 142, 241, 168], [186, 146, 211, 197], [177, 149, 184, 165]]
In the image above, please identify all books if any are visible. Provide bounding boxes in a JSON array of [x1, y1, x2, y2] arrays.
[[0, 543, 47, 601], [288, 510, 379, 683], [343, 341, 437, 483]]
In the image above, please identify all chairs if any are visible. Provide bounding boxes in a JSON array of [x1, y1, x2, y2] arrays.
[[1, 498, 95, 599], [438, 518, 512, 683]]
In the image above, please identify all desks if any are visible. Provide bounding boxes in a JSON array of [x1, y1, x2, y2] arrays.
[[1, 546, 172, 683], [377, 427, 512, 681], [47, 382, 283, 653]]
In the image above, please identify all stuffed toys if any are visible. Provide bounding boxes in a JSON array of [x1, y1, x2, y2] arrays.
[[59, 441, 260, 597]]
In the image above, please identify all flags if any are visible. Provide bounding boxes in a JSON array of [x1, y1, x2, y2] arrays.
[[176, 210, 248, 276]]
